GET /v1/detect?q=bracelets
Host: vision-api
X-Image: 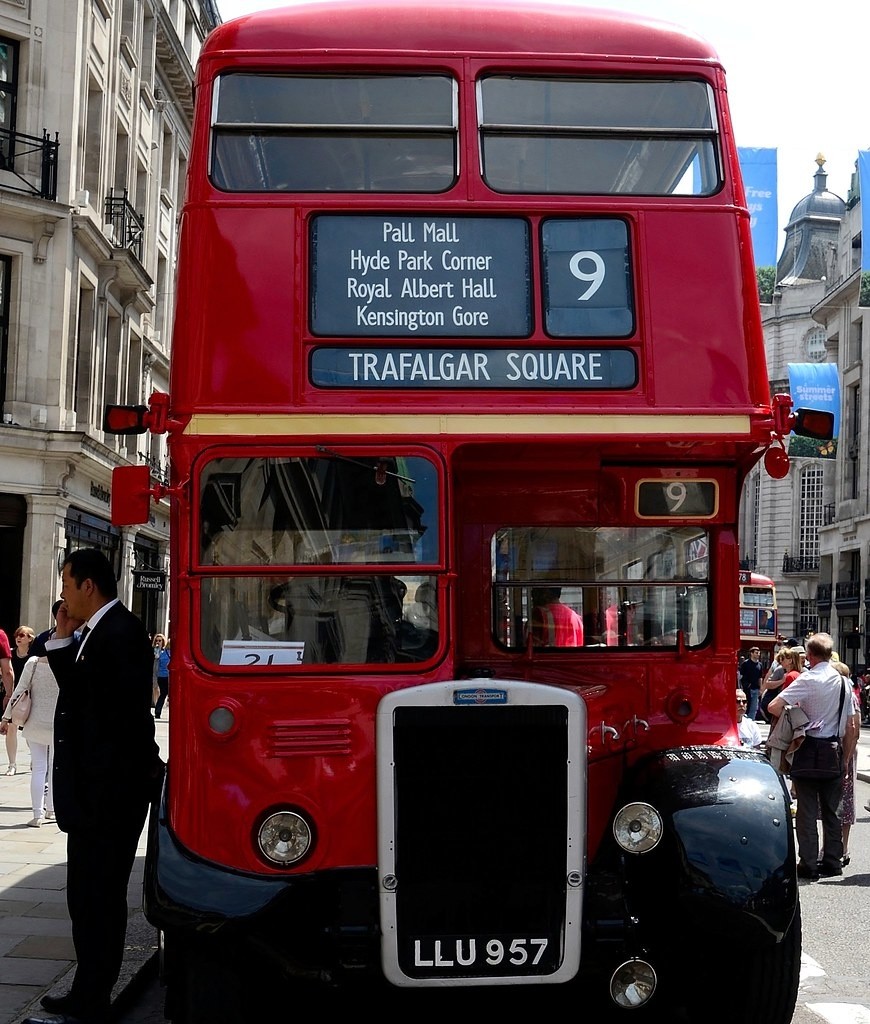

[6,694,11,698]
[2,719,9,722]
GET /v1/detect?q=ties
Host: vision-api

[74,625,91,661]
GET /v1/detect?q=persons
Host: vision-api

[523,588,583,646]
[607,589,635,645]
[0,600,83,827]
[404,583,438,632]
[40,547,170,1014]
[735,633,870,878]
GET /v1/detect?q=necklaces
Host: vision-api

[18,648,28,658]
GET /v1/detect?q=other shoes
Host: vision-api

[796,863,820,881]
[26,819,42,828]
[155,715,160,719]
[45,812,56,820]
[6,763,17,775]
[815,862,843,877]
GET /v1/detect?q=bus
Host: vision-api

[691,568,782,679]
[104,0,837,1024]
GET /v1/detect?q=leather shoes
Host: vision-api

[22,1017,68,1024]
[40,996,69,1015]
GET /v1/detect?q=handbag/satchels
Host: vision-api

[10,657,40,725]
[789,737,843,781]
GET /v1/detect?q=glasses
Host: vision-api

[14,632,29,638]
[736,700,748,705]
[777,657,785,662]
[155,640,163,642]
